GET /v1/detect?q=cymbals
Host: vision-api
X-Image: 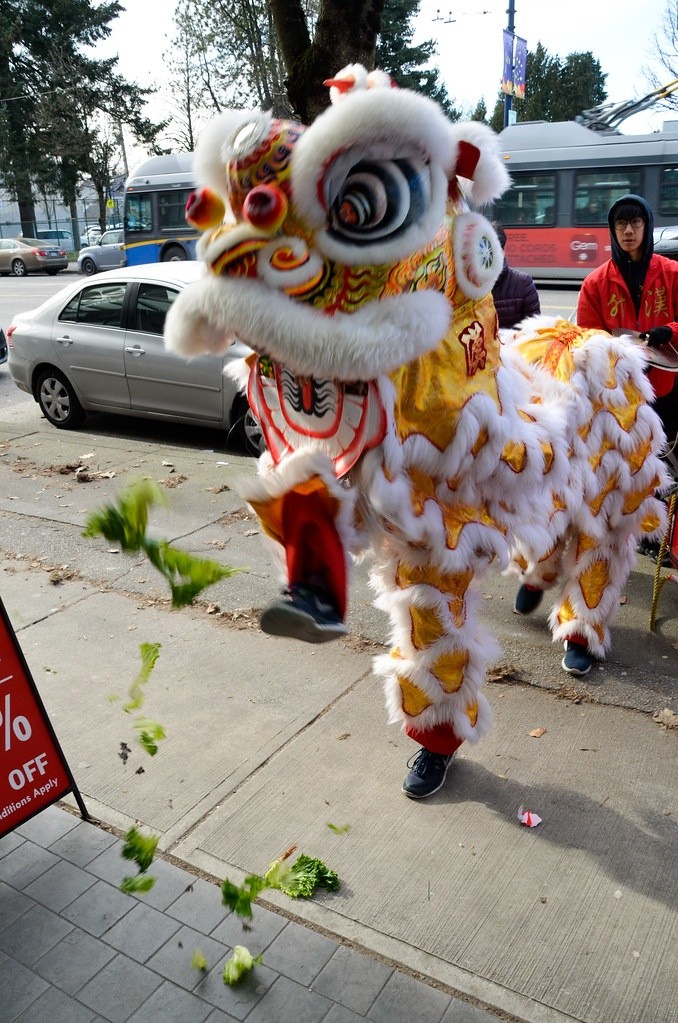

[612,327,677,373]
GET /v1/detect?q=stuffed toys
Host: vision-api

[160,64,674,807]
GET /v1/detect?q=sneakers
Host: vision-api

[402,745,459,799]
[515,584,544,616]
[262,592,347,644]
[561,639,591,675]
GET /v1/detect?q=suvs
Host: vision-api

[77,229,126,275]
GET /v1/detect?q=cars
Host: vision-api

[0,237,69,277]
[6,261,271,460]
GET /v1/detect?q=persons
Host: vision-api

[575,193,678,569]
[490,218,541,331]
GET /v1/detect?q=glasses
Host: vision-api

[614,219,645,231]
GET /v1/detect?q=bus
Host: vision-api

[476,117,677,290]
[123,150,203,265]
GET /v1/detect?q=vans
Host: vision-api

[19,229,89,252]
